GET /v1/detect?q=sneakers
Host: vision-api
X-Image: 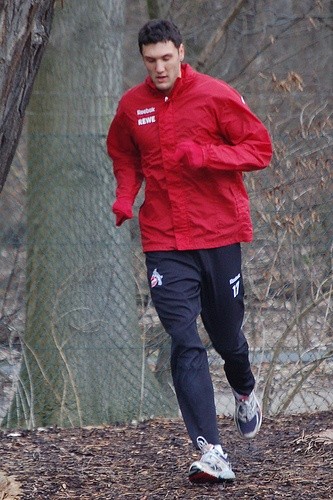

[189,436,235,482]
[230,385,263,440]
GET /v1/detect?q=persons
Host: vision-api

[106,20,274,480]
